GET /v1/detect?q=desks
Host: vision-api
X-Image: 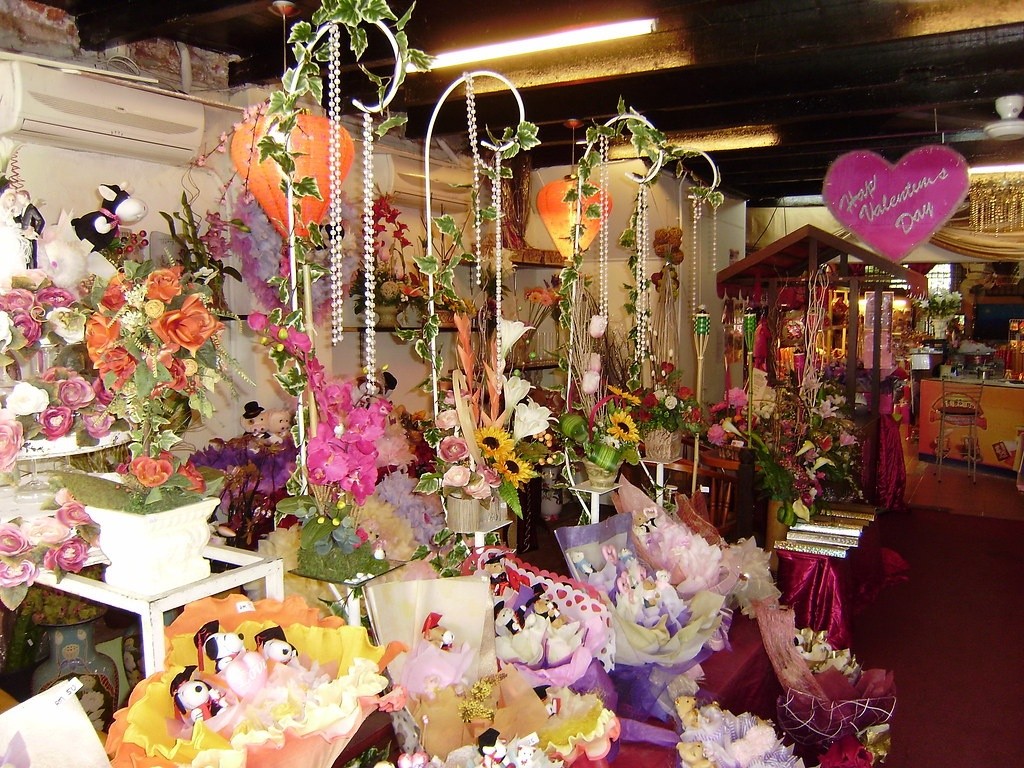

[917,373,1024,472]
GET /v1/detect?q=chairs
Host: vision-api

[933,374,985,484]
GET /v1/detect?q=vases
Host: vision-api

[446,496,481,533]
[121,607,181,690]
[30,608,119,736]
[644,425,680,461]
[930,317,949,337]
[83,496,222,598]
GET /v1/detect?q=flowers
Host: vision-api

[0,184,963,768]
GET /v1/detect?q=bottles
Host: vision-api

[974,348,981,366]
[984,353,991,365]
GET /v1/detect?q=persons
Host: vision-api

[1,190,45,271]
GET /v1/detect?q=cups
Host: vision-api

[983,372,989,379]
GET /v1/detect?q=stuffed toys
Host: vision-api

[676,696,720,768]
[573,546,671,605]
[792,628,847,672]
[422,613,454,652]
[71,182,148,252]
[356,371,397,409]
[169,618,299,725]
[485,554,565,635]
[476,728,538,768]
[239,401,294,443]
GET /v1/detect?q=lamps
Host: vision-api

[227,1,357,253]
[402,16,660,77]
[535,119,612,261]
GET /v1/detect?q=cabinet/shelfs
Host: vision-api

[31,540,284,679]
[327,253,570,379]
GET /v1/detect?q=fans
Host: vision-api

[895,96,1023,142]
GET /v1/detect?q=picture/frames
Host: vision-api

[582,456,623,487]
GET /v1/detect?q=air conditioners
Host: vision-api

[0,60,206,168]
